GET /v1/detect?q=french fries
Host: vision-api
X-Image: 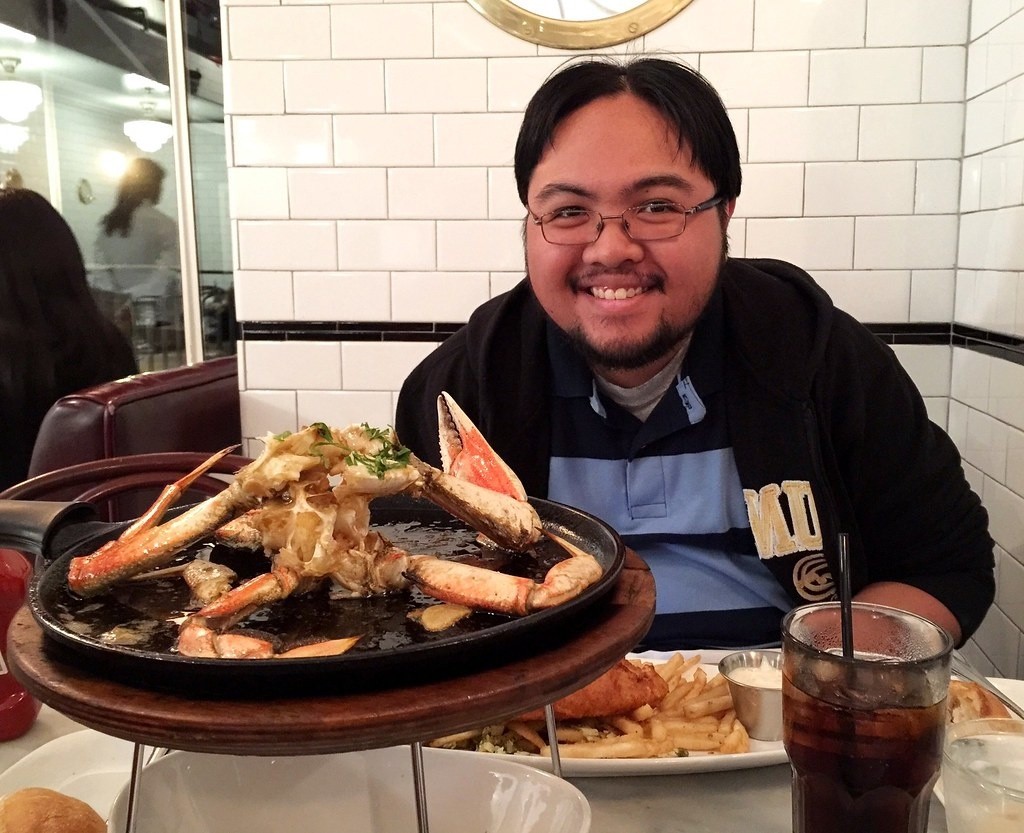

[433,653,754,759]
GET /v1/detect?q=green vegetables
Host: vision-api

[274,422,411,481]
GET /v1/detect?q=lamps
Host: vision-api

[0,56,43,123]
[123,103,173,153]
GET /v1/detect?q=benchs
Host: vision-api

[27,353,243,524]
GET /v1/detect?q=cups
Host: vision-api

[780,599,955,833]
[939,716,1024,833]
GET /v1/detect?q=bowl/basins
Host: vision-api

[718,650,785,742]
[107,745,592,833]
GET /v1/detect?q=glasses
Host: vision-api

[530,196,723,245]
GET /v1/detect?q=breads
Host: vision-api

[942,678,1019,735]
[0,787,108,833]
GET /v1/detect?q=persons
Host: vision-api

[1,186,140,487]
[92,159,179,323]
[396,59,996,663]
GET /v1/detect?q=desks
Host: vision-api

[0,675,1023,833]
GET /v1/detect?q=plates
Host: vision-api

[464,656,790,778]
[0,728,177,833]
[28,495,627,704]
[949,675,1024,721]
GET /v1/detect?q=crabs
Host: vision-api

[64,388,604,660]
